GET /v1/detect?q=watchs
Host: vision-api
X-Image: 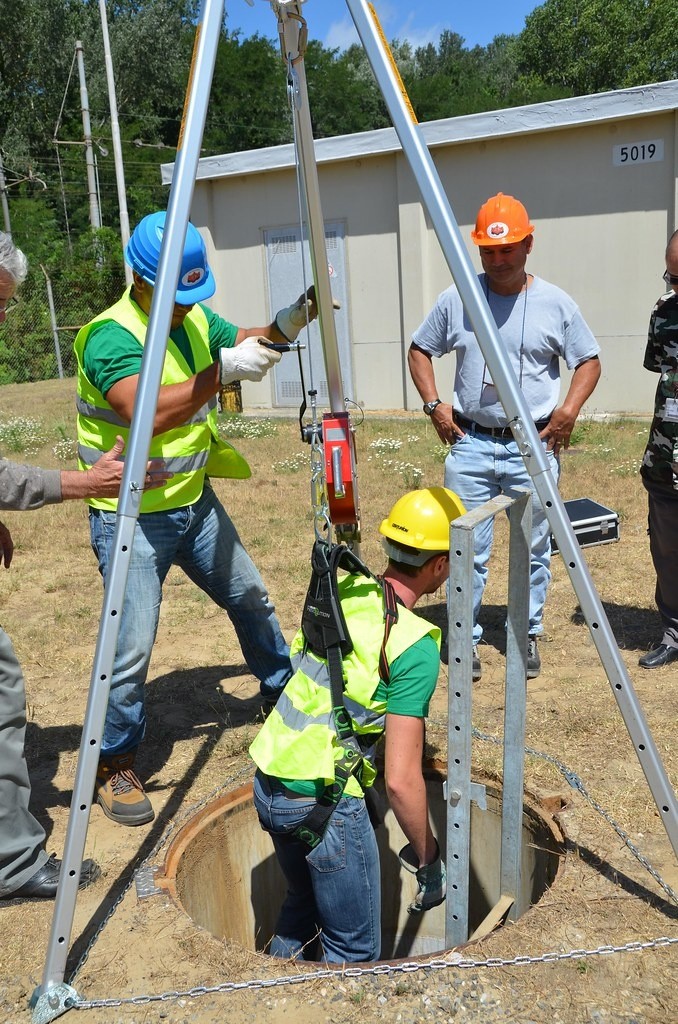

[423,399,442,415]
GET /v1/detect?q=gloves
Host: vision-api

[399,836,446,914]
[275,284,340,343]
[219,335,283,385]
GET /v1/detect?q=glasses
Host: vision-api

[482,343,524,387]
[0,296,19,314]
[663,269,678,285]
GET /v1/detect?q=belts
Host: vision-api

[453,413,550,438]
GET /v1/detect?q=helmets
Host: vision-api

[124,211,216,306]
[471,192,535,247]
[380,487,476,554]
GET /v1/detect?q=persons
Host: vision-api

[246,489,470,968]
[406,191,601,679]
[0,227,174,909]
[71,212,343,826]
[638,230,678,669]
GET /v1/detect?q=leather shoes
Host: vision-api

[639,643,678,668]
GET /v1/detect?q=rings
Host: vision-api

[556,441,561,445]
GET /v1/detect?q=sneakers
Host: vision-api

[93,745,155,827]
[526,634,541,678]
[0,853,101,907]
[262,700,277,716]
[472,652,482,678]
